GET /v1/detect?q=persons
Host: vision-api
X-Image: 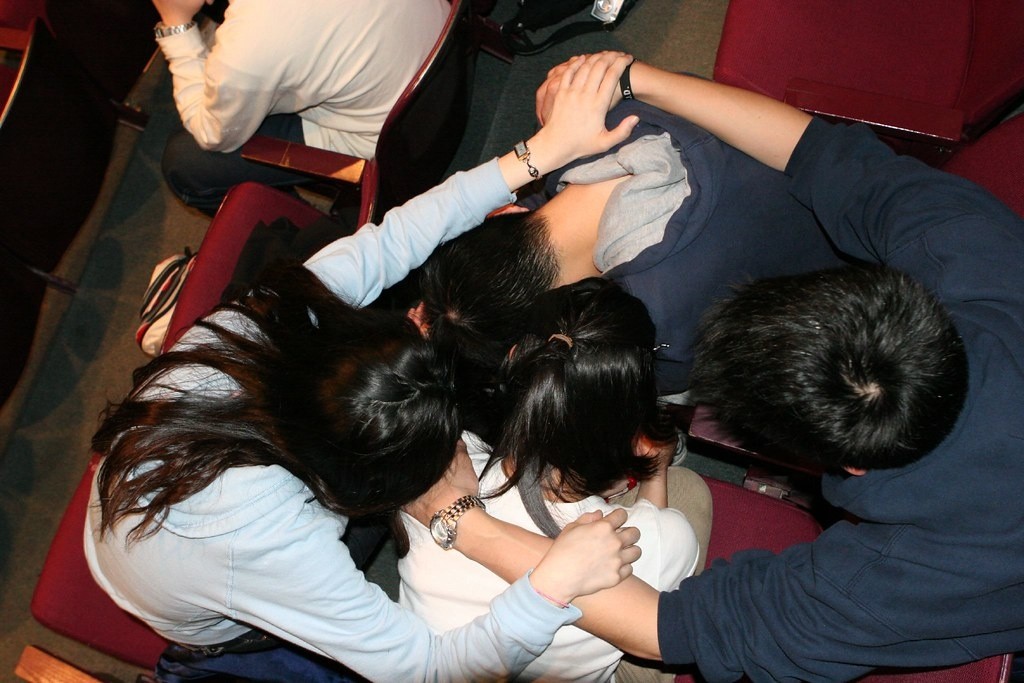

[152,1,451,220]
[83,49,1024,683]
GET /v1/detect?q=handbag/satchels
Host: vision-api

[135,244,203,359]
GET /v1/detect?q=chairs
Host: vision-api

[1,1,1024,683]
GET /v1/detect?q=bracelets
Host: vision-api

[619,58,638,101]
[536,589,571,608]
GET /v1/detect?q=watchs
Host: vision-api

[154,22,195,38]
[430,494,487,550]
[514,140,542,181]
